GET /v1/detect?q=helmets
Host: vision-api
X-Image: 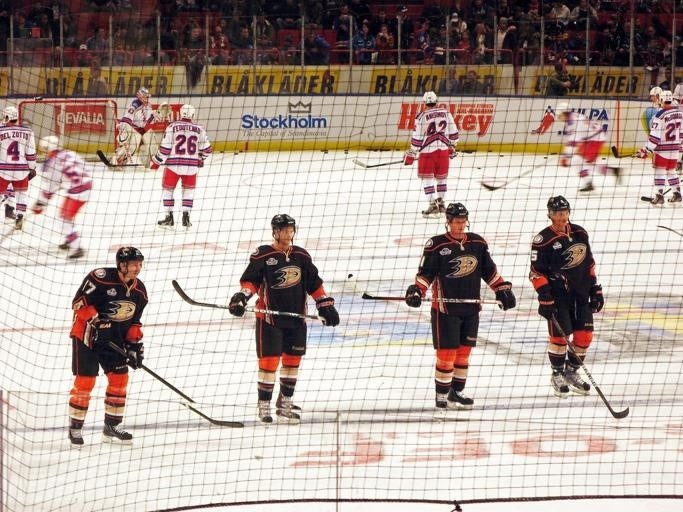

[135,86,151,107]
[1,105,19,124]
[116,246,144,274]
[554,102,574,120]
[650,86,673,106]
[445,202,469,223]
[422,91,438,104]
[546,195,570,211]
[39,136,63,152]
[271,213,296,244]
[180,104,196,119]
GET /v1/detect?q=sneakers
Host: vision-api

[69,428,84,444]
[550,366,569,392]
[448,388,473,405]
[258,400,272,423]
[103,423,132,440]
[563,362,590,391]
[614,167,623,184]
[182,212,192,226]
[435,392,448,407]
[579,182,594,192]
[650,186,682,204]
[276,392,300,409]
[5,204,23,230]
[158,212,174,226]
[59,241,84,258]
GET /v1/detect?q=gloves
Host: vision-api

[405,284,422,307]
[229,292,246,316]
[124,340,144,370]
[402,149,416,165]
[31,200,47,214]
[493,281,516,311]
[91,321,113,347]
[538,292,559,319]
[589,284,604,312]
[150,163,160,169]
[316,297,339,327]
[449,145,456,159]
[633,146,653,159]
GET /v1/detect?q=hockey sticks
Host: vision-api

[352,159,420,168]
[642,188,671,202]
[611,146,633,158]
[107,340,243,427]
[348,273,503,304]
[172,280,326,321]
[96,150,146,167]
[479,155,559,191]
[551,312,630,418]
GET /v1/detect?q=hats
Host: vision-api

[79,44,88,50]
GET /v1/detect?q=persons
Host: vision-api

[1,183,17,220]
[229,213,340,414]
[554,101,624,193]
[631,70,683,203]
[1,0,682,71]
[437,69,458,93]
[29,134,93,260]
[63,245,149,447]
[0,105,37,230]
[526,195,606,393]
[111,85,153,172]
[542,60,572,97]
[462,71,484,94]
[404,202,517,410]
[401,90,461,214]
[148,103,213,228]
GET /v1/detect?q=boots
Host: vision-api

[422,198,446,215]
